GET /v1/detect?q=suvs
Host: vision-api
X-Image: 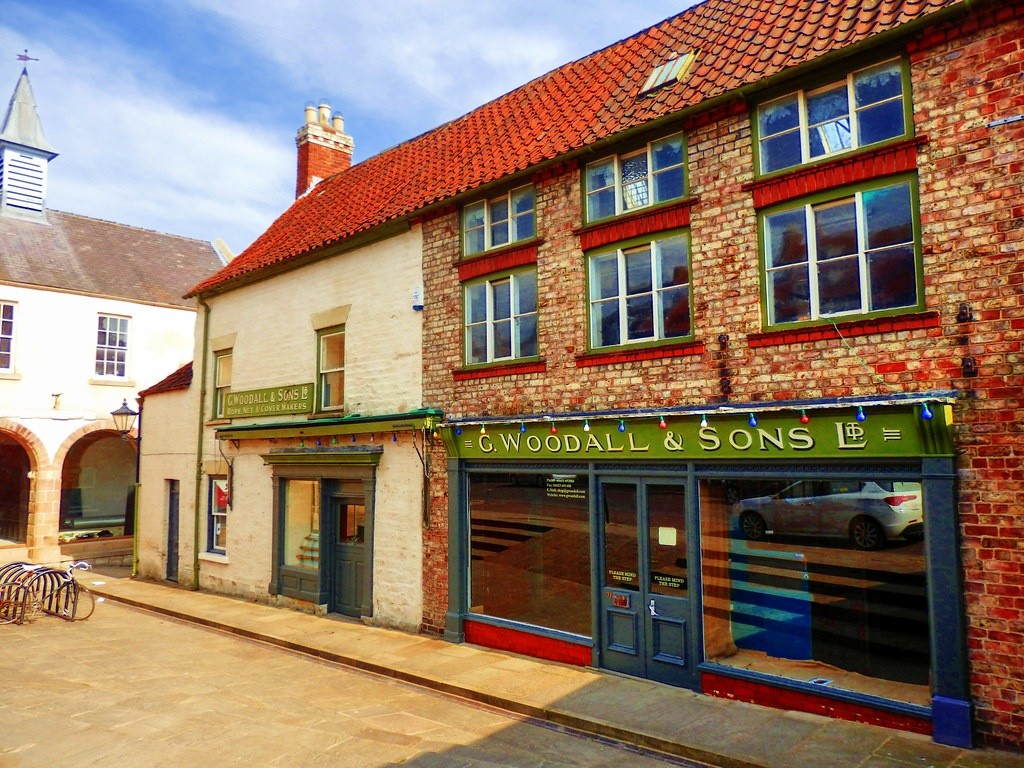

[728,478,924,552]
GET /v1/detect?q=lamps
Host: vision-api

[112,399,142,443]
